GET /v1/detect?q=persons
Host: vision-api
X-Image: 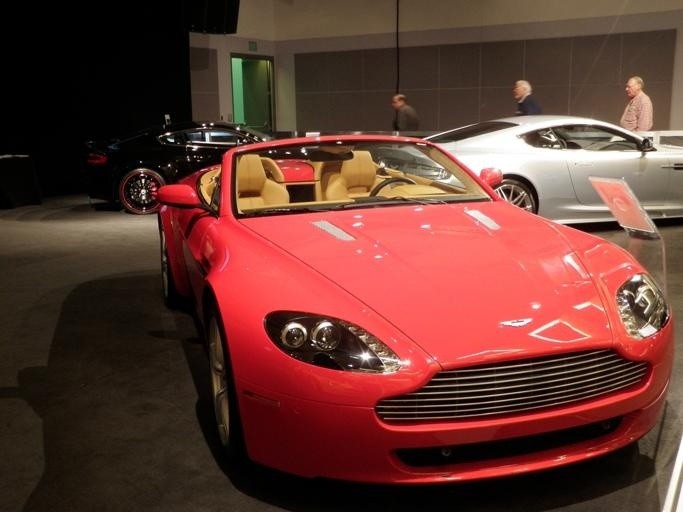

[620,76,655,132]
[513,80,543,116]
[391,94,420,131]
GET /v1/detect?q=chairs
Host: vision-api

[202,152,289,209]
[322,150,394,201]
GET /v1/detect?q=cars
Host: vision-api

[72,119,283,219]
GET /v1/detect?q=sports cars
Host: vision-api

[416,112,683,231]
[149,132,674,491]
